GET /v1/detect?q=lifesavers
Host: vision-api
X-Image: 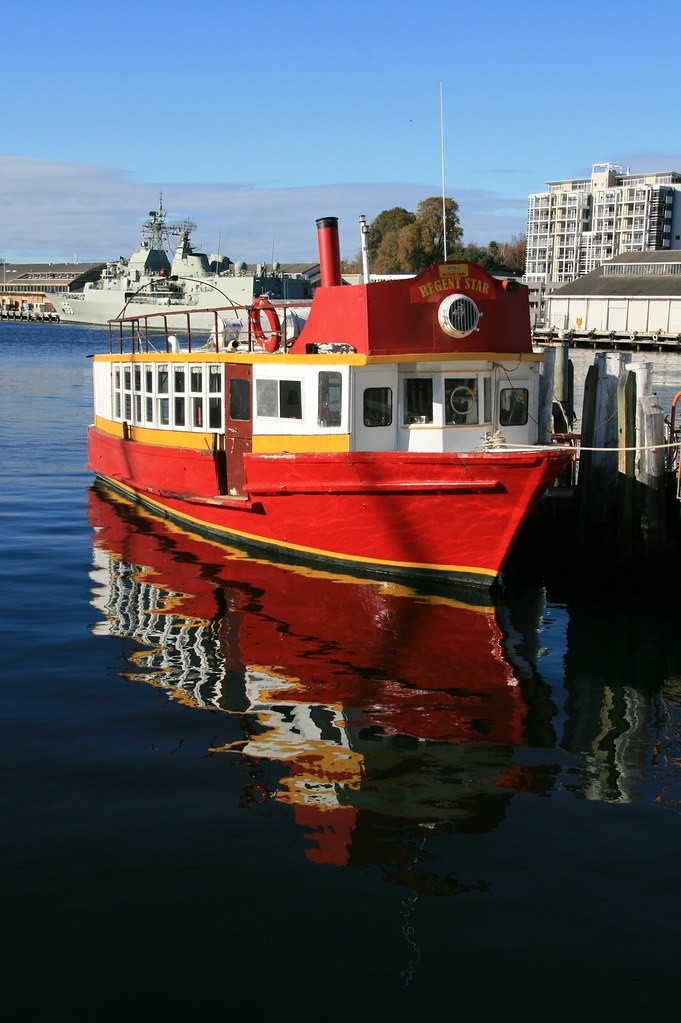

[249,295,283,353]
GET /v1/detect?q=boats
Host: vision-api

[82,86,577,589]
[43,189,291,335]
[87,475,564,866]
